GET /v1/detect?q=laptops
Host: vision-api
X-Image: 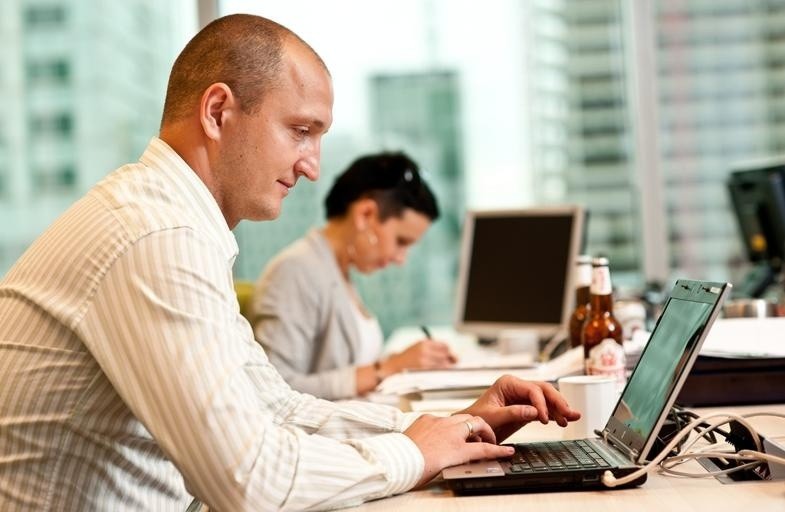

[440,278,733,497]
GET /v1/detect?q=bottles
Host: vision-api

[569,254,626,381]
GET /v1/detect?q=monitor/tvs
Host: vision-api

[727,166,785,302]
[449,205,583,358]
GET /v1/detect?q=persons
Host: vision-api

[243,148,462,405]
[1,12,584,511]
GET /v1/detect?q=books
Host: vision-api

[368,321,583,398]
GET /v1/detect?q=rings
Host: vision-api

[463,419,475,440]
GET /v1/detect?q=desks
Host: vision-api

[194,358,785,511]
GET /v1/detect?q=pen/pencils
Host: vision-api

[422,326,432,340]
